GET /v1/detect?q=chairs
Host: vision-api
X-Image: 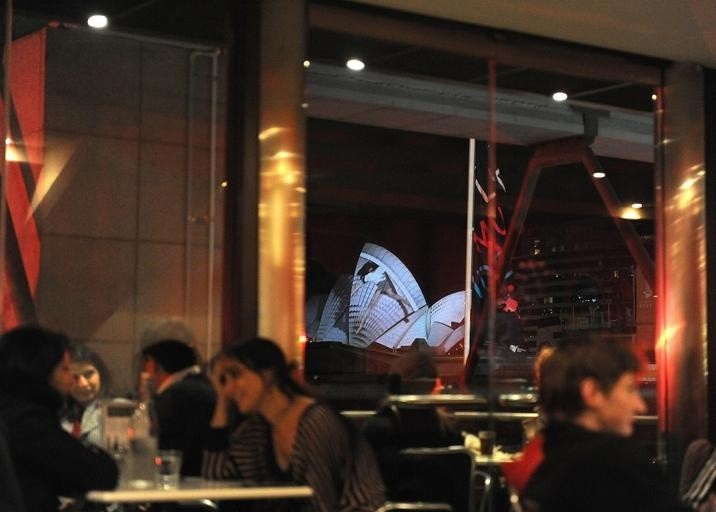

[376,447,490,511]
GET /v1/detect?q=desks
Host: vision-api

[82,478,317,511]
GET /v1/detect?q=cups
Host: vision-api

[153,454,181,489]
[477,430,498,456]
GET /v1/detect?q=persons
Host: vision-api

[194,332,387,510]
[353,350,469,501]
[516,331,673,512]
[57,338,110,446]
[495,344,564,492]
[0,323,118,510]
[136,317,249,481]
